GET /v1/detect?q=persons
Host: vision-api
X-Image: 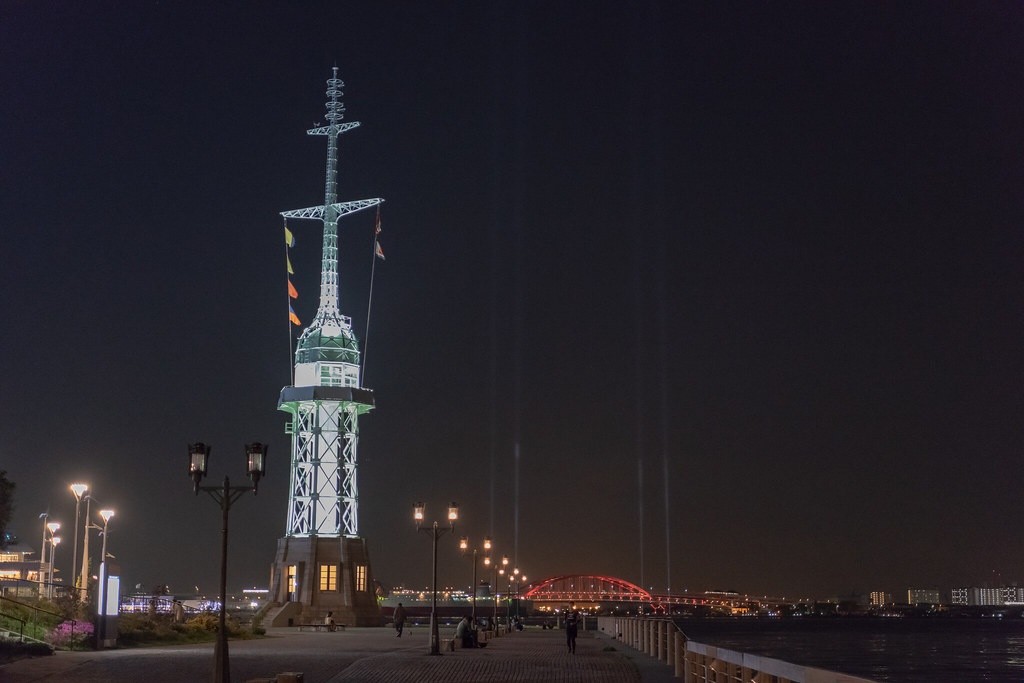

[393,602,407,638]
[170,599,183,622]
[325,611,336,632]
[456,616,481,648]
[563,601,581,655]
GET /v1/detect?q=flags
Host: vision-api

[288,281,298,298]
[288,260,294,274]
[285,228,292,248]
[289,305,301,325]
[375,241,385,258]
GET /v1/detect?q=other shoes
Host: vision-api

[568,648,571,653]
[396,633,402,639]
[331,630,337,632]
[572,651,575,655]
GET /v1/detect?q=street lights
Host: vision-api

[413,501,459,656]
[70,484,88,599]
[510,575,527,627]
[97,510,115,651]
[187,440,269,683]
[460,535,491,649]
[498,568,519,633]
[47,523,61,601]
[483,553,509,639]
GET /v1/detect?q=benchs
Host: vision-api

[439,624,508,652]
[298,623,347,632]
